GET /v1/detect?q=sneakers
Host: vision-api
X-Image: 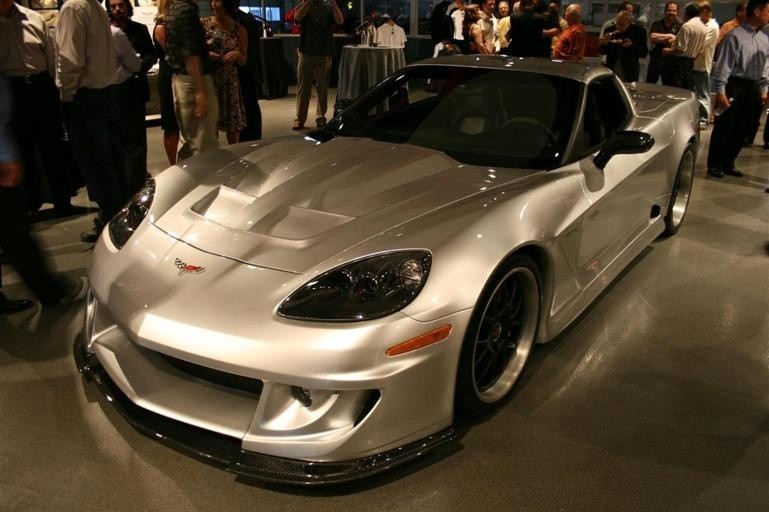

[61,274,89,306]
[293,120,304,129]
[80,216,102,242]
[316,118,326,128]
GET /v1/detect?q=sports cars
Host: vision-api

[72,51,704,489]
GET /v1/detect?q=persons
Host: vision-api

[599,2,648,83]
[293,1,344,129]
[646,2,719,129]
[1,1,157,316]
[425,1,587,92]
[708,1,769,177]
[153,0,262,165]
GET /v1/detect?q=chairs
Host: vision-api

[439,73,604,146]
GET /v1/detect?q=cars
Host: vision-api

[142,60,162,115]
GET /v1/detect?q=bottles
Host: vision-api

[367,20,378,45]
[361,30,367,45]
[353,31,360,46]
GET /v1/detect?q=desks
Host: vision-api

[336,44,406,116]
[259,36,289,99]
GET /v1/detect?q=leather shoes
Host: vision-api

[707,165,724,179]
[725,167,744,178]
[1,293,33,313]
